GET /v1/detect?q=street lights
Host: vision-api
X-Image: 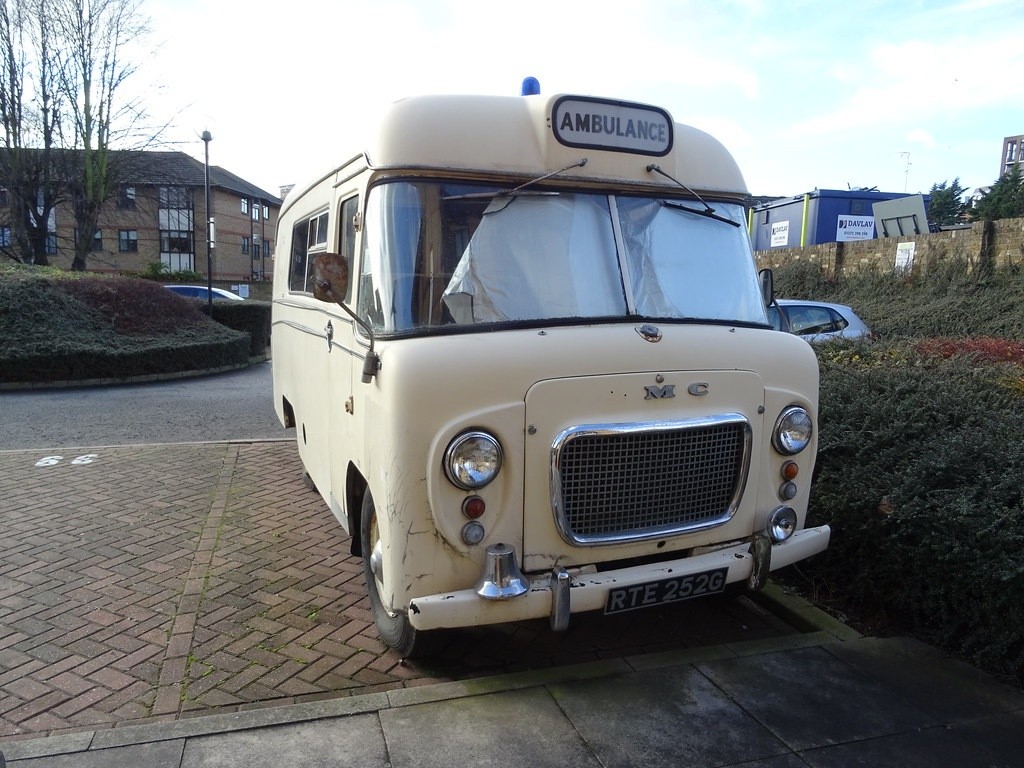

[201,131,213,319]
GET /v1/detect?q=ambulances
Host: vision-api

[269,76,832,662]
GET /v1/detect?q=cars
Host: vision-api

[765,299,873,344]
[164,285,244,301]
